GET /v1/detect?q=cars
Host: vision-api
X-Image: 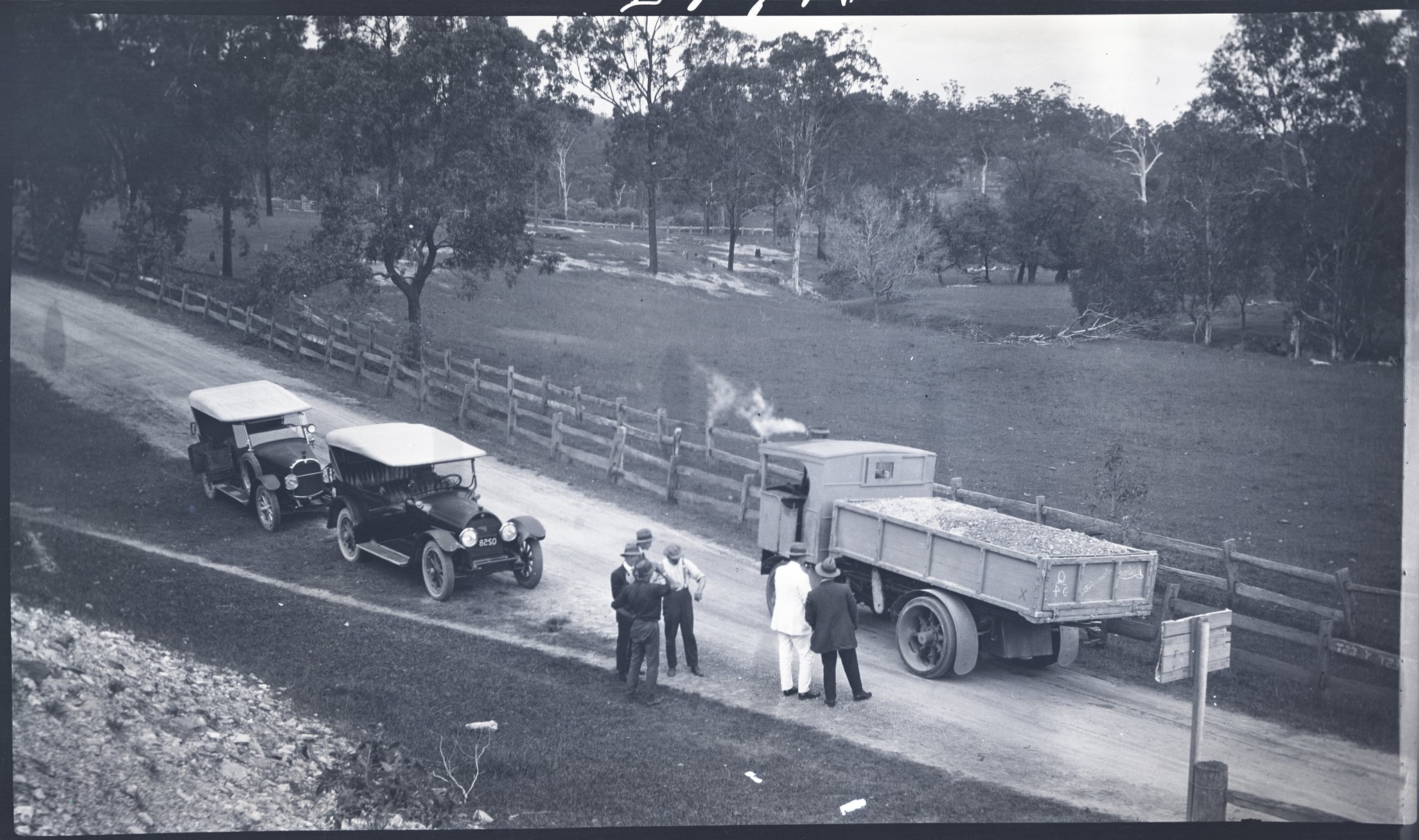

[323,423,546,602]
[190,379,336,531]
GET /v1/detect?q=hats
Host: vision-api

[633,561,658,573]
[635,528,656,541]
[622,543,643,556]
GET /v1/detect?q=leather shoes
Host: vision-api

[814,556,842,577]
[854,691,872,700]
[824,698,835,706]
[663,543,686,560]
[798,690,820,700]
[784,687,799,696]
[638,670,645,674]
[691,666,704,676]
[648,697,665,705]
[619,675,628,682]
[667,666,676,676]
[788,542,812,556]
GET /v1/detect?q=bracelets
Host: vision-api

[662,572,668,577]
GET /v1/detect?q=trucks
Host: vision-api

[758,426,1162,680]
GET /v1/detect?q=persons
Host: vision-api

[610,560,679,705]
[609,543,646,681]
[633,528,658,662]
[656,544,707,676]
[768,542,820,700]
[804,556,872,707]
